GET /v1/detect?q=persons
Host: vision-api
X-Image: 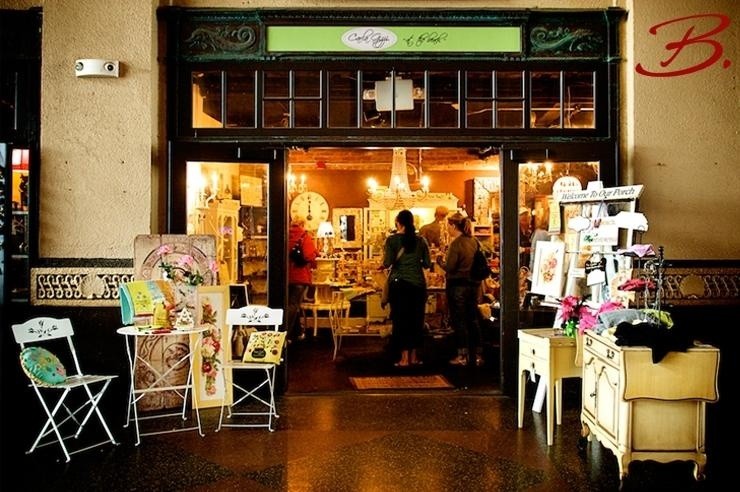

[288,214,321,344]
[436,213,492,368]
[519,207,532,271]
[529,211,551,273]
[419,206,449,247]
[383,210,431,369]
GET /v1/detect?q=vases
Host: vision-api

[562,318,577,337]
[173,303,195,331]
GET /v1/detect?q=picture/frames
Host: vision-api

[331,207,363,250]
[239,174,264,208]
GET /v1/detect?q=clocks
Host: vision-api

[290,191,331,232]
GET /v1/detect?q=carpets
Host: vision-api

[348,374,455,391]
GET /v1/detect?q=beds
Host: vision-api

[329,301,454,360]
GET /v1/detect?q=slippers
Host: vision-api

[394,362,410,367]
[411,361,424,365]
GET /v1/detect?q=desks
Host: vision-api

[116,325,213,447]
[299,302,351,341]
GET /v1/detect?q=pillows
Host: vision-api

[18,346,67,386]
[241,330,287,365]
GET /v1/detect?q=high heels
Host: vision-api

[450,355,468,366]
[475,356,485,366]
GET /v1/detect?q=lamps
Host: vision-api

[315,221,335,258]
[364,148,431,212]
[519,160,554,199]
[287,165,307,194]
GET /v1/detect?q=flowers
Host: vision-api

[155,226,235,305]
[556,293,592,335]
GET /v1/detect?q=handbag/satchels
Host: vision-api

[471,238,492,281]
[289,231,310,268]
[382,247,404,309]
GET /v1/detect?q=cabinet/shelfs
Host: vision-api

[574,323,720,492]
[366,291,392,320]
[464,175,525,225]
[471,224,494,260]
[196,204,239,285]
[516,326,585,447]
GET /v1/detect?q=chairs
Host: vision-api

[213,304,284,435]
[10,316,121,465]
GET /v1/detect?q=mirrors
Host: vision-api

[364,192,461,261]
